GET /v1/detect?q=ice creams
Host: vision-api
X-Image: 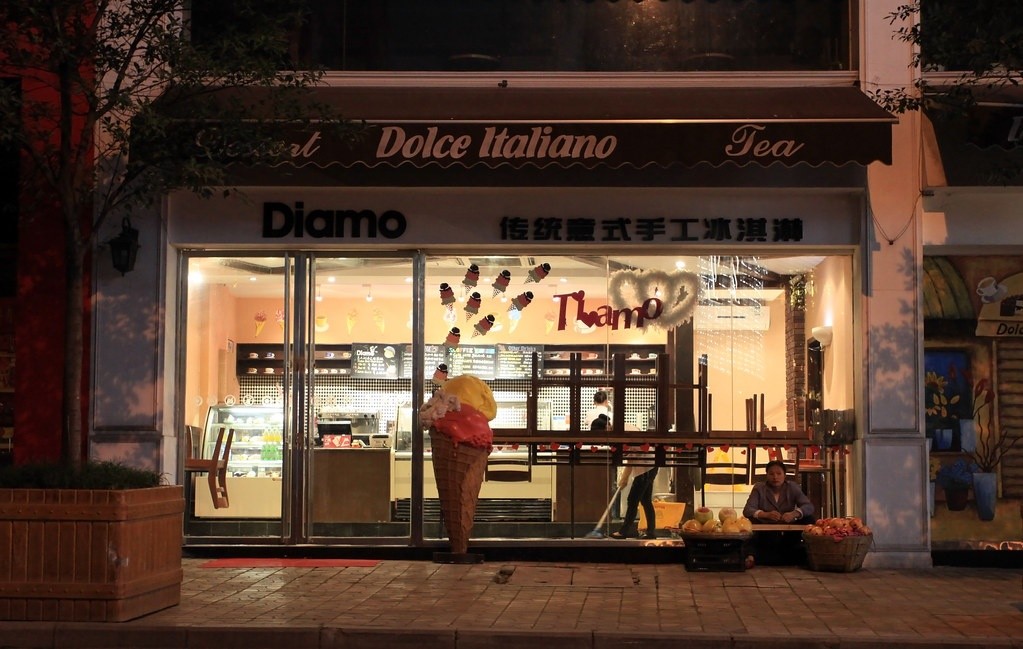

[419,374,497,554]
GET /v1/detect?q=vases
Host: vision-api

[935,428,952,450]
[927,437,933,452]
[974,472,999,522]
[929,481,935,516]
[944,485,965,510]
[958,418,977,452]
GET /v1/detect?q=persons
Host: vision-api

[586,391,613,426]
[743,461,815,569]
[591,414,658,538]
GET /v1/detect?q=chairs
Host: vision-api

[184,426,235,510]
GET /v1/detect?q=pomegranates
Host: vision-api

[804,517,871,535]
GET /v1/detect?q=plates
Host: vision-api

[265,357,274,358]
[981,285,1008,304]
[316,325,329,332]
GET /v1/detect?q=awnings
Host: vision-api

[126,86,899,166]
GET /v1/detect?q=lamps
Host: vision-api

[109,214,140,277]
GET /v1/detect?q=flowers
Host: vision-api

[925,370,1023,490]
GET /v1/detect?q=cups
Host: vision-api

[588,353,598,357]
[314,369,347,373]
[265,367,274,372]
[266,352,275,357]
[586,369,603,374]
[976,277,999,297]
[326,353,334,357]
[250,353,258,357]
[632,369,641,373]
[629,353,657,359]
[249,368,257,372]
[316,316,327,326]
[343,353,351,357]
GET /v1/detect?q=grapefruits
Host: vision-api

[682,506,752,533]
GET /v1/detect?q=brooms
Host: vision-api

[583,486,622,539]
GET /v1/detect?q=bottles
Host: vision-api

[274,426,281,442]
[262,443,283,460]
[263,426,269,442]
[269,427,274,442]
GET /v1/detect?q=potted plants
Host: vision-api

[1,454,184,623]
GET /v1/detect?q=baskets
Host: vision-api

[799,531,874,572]
[676,529,756,572]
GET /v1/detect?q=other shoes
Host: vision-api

[638,533,657,539]
[604,532,626,539]
